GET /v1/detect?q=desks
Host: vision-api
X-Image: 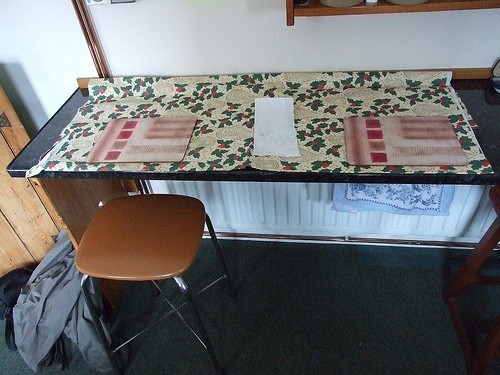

[8,79,500,316]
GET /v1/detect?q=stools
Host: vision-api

[74,195,236,375]
[443,183,500,375]
